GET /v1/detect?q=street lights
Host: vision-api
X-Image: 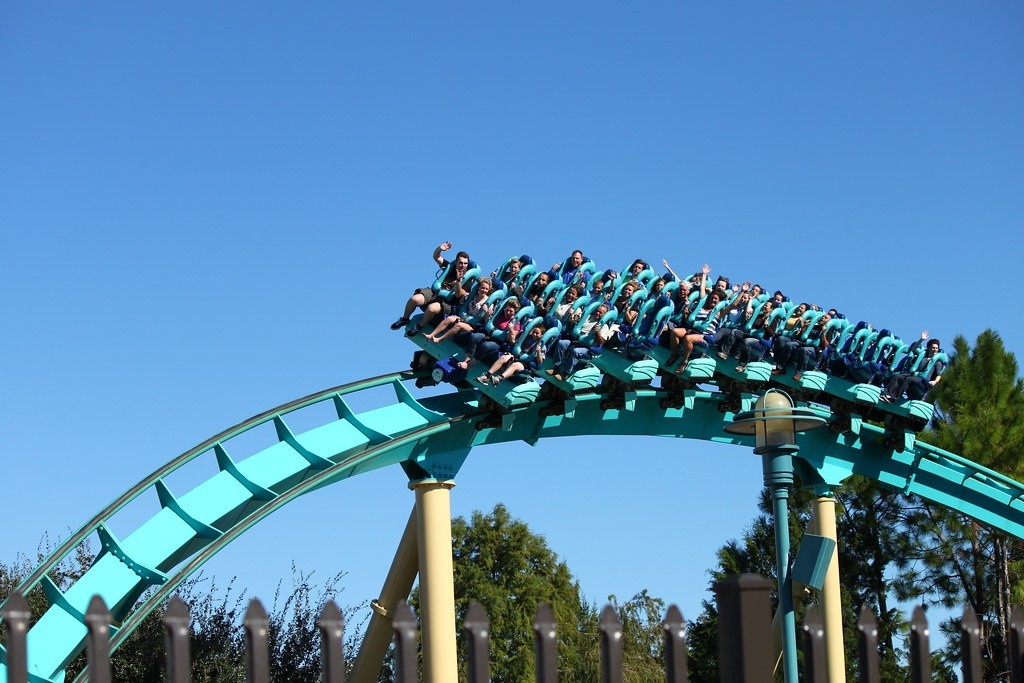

[721,386,836,683]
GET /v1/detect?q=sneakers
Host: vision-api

[407,325,421,337]
[391,317,410,330]
[491,375,499,387]
[476,375,490,386]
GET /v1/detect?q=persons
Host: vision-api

[390,241,950,406]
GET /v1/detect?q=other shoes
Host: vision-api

[554,373,568,381]
[735,354,741,360]
[675,361,688,374]
[793,374,800,381]
[545,367,560,375]
[458,358,471,371]
[879,393,896,404]
[735,365,746,373]
[716,352,727,360]
[665,353,680,367]
[772,367,785,375]
